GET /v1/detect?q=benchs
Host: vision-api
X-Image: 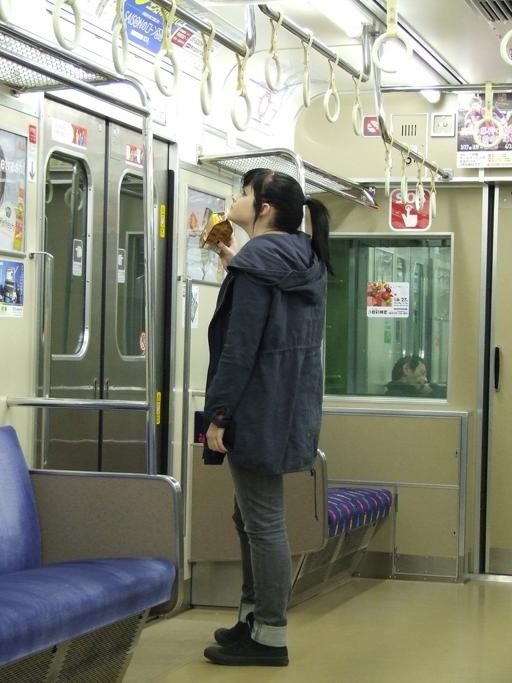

[193,411,392,610]
[0,427,184,683]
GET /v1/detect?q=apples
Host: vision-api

[381,291,393,306]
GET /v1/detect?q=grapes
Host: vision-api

[384,284,391,292]
[367,282,384,306]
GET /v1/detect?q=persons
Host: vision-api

[385,355,440,397]
[202,167,336,668]
[465,97,495,141]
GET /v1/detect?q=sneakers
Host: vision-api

[213,622,250,646]
[204,640,289,666]
